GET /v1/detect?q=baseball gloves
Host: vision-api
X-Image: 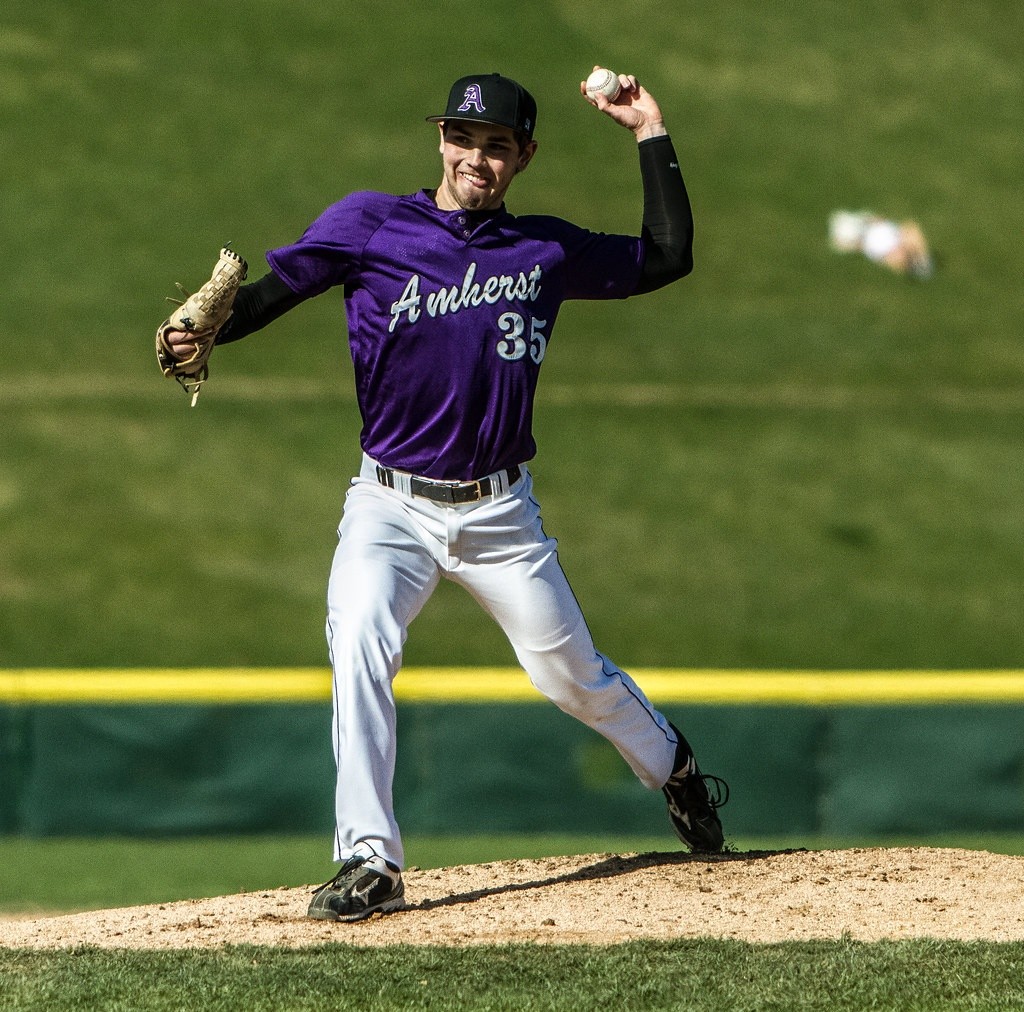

[154,243,250,408]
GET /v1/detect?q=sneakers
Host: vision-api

[662,721,730,855]
[307,841,407,922]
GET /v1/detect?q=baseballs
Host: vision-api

[585,68,622,104]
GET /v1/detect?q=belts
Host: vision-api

[377,465,522,503]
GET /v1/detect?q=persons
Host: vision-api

[830,207,933,276]
[156,65,730,921]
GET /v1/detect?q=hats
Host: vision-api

[426,72,537,142]
